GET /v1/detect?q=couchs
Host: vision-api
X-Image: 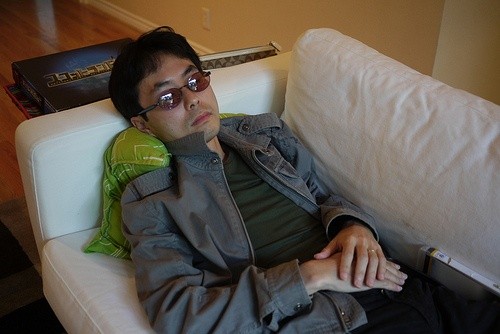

[15,28,500,334]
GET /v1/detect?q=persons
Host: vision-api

[108,25,500,334]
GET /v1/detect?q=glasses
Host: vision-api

[137,71,211,116]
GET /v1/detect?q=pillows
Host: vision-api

[83,113,257,262]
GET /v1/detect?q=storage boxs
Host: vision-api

[11,37,136,113]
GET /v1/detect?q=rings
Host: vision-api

[368,248,376,252]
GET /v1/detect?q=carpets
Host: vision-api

[0,222,70,334]
